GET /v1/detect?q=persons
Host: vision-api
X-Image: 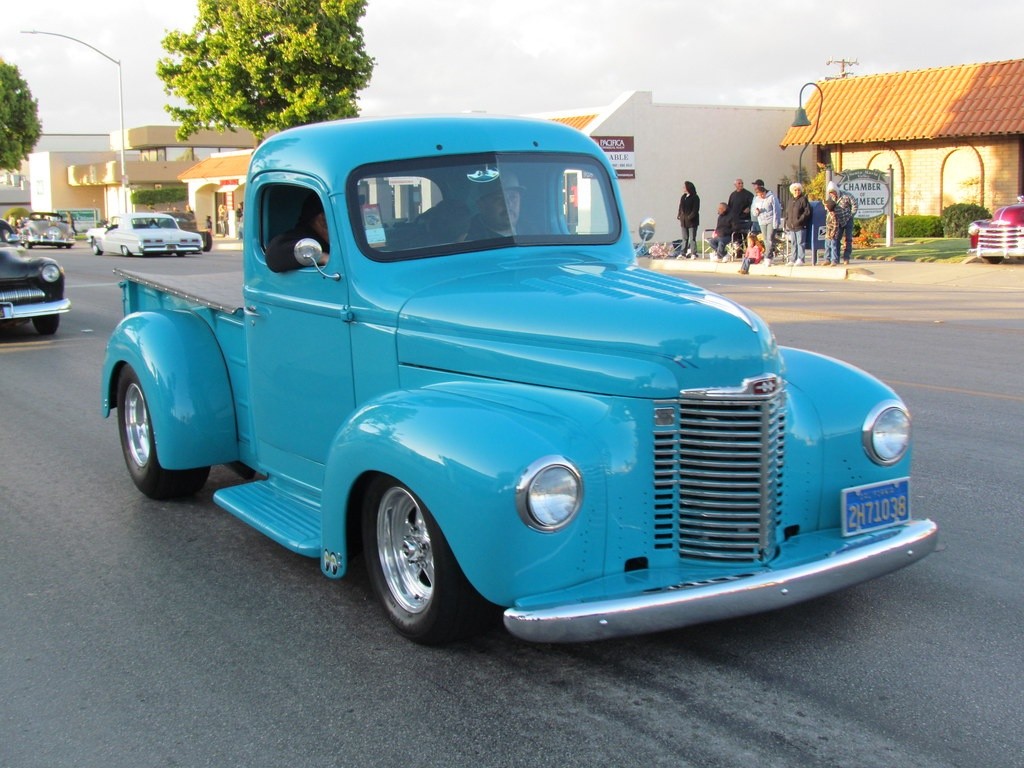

[826,181,858,265]
[783,183,812,266]
[66,210,77,236]
[146,201,244,241]
[737,236,761,274]
[727,178,754,258]
[264,186,366,273]
[754,187,781,267]
[749,178,765,235]
[708,201,735,263]
[676,181,700,260]
[459,167,528,242]
[820,200,842,266]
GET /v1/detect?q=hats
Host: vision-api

[752,179,764,186]
[824,200,836,211]
[479,170,527,201]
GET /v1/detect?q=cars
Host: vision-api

[967,193,1024,263]
[18,212,78,248]
[0,219,72,334]
[85,213,205,256]
[160,211,212,251]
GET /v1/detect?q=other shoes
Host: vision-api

[764,258,773,267]
[739,269,748,274]
[844,258,849,265]
[722,253,732,262]
[785,259,804,266]
[691,255,696,260]
[677,254,686,259]
[821,260,837,266]
[710,255,720,261]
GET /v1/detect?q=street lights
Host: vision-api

[791,82,824,183]
[19,29,126,210]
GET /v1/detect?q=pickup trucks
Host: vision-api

[101,110,935,644]
